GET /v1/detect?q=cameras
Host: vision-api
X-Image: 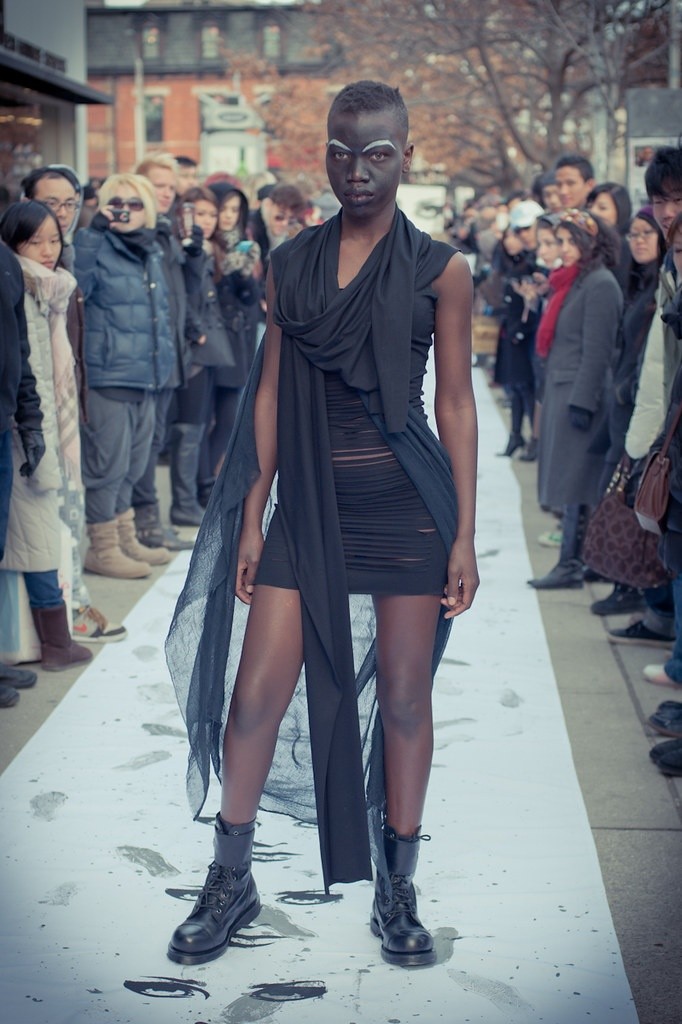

[108,210,130,223]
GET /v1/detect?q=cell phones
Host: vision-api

[231,241,253,254]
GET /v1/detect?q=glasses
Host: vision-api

[40,198,77,213]
[624,228,657,240]
[274,212,298,227]
[108,196,145,211]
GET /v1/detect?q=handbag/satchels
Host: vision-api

[581,449,667,589]
[634,452,670,536]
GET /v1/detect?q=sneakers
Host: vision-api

[72,605,127,642]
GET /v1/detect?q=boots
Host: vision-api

[33,602,92,672]
[82,508,171,578]
[168,809,260,963]
[170,422,206,525]
[370,821,436,966]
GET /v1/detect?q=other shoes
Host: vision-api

[503,431,524,455]
[643,664,677,687]
[1,665,37,706]
[538,530,563,548]
[518,437,538,462]
[527,568,583,589]
[138,523,194,551]
[591,594,647,615]
[649,738,681,777]
[648,699,682,738]
[608,621,676,648]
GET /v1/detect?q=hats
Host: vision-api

[510,201,545,230]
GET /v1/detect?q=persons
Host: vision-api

[166,81,481,967]
[0,156,343,711]
[443,148,682,774]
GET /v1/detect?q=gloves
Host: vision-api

[18,428,47,477]
[568,406,592,431]
